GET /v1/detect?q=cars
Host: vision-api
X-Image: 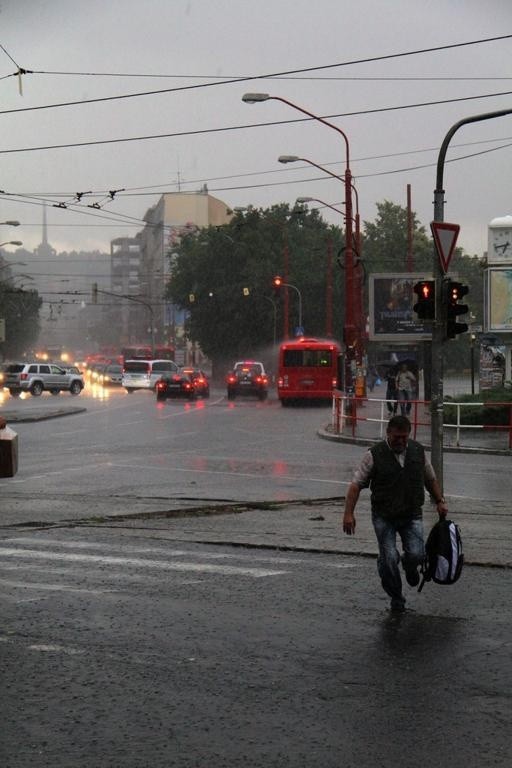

[0,359,85,397]
[227,367,265,400]
[156,366,210,403]
[73,355,124,387]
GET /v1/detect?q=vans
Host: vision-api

[121,358,181,393]
[234,362,268,396]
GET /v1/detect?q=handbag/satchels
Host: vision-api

[427,520,463,585]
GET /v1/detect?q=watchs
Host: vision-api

[436,495,446,504]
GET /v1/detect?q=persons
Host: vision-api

[0,415,8,430]
[342,414,449,611]
[365,358,417,416]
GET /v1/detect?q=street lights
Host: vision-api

[277,155,366,410]
[272,278,304,337]
[240,93,360,429]
[295,196,369,403]
[241,291,277,385]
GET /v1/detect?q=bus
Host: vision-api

[121,343,176,361]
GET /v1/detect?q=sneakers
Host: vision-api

[390,597,406,613]
[401,553,419,586]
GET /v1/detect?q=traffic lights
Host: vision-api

[444,278,471,341]
[411,277,436,322]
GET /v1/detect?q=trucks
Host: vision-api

[44,342,62,359]
